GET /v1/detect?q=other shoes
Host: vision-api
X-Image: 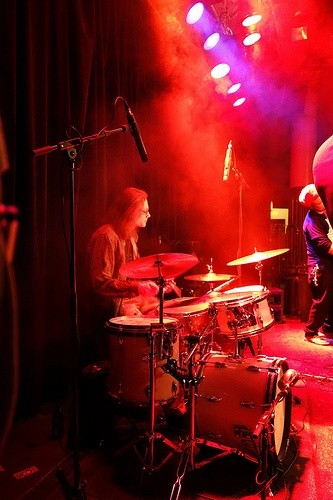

[304,330,333,346]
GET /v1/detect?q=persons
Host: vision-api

[298,185,333,345]
[88,188,178,379]
[312,134,333,226]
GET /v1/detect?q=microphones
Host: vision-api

[122,101,148,163]
[222,144,232,182]
[279,367,297,390]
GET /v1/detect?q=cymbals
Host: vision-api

[184,274,239,281]
[119,253,198,279]
[227,247,290,266]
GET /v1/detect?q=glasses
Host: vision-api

[139,209,150,215]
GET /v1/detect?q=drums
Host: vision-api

[155,296,216,337]
[211,284,275,340]
[194,350,292,466]
[104,315,180,404]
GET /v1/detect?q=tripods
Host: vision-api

[125,279,223,488]
